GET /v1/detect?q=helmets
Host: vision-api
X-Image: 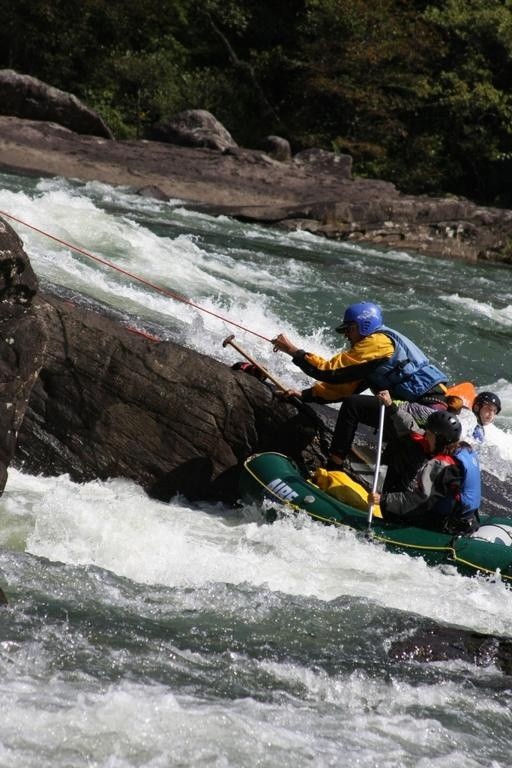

[472,392,501,414]
[425,411,461,446]
[335,303,383,336]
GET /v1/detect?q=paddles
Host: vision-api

[223,334,376,473]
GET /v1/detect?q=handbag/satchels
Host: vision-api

[447,396,463,414]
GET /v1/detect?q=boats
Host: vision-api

[241,450,512,586]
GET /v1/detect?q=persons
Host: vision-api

[367,387,484,540]
[472,389,502,443]
[270,298,454,495]
